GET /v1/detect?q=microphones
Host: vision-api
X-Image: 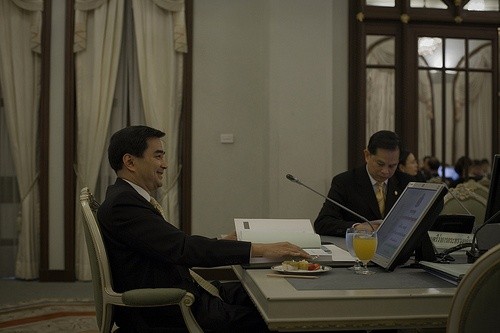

[439,182,471,215]
[286,174,375,231]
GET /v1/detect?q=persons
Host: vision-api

[314,130,427,239]
[395,148,492,188]
[96,125,311,333]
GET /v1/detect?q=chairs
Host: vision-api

[80,187,205,333]
[441,178,491,232]
[446,245,500,333]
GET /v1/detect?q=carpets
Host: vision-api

[0,296,119,333]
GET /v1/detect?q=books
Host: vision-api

[232,216,332,270]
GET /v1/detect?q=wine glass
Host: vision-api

[345,227,371,273]
[352,229,378,274]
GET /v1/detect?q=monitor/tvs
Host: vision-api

[438,165,459,180]
[371,181,448,272]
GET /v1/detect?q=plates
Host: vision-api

[270,265,333,273]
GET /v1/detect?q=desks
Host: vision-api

[219,234,457,333]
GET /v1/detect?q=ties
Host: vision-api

[150,196,223,301]
[375,182,385,219]
